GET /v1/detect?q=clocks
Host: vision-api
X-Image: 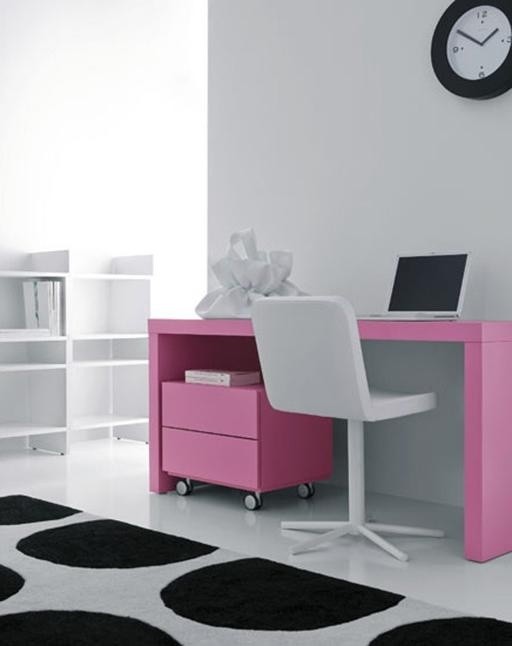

[430,0,512,100]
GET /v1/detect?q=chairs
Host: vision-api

[249,296,446,564]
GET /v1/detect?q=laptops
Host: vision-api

[356,250,471,321]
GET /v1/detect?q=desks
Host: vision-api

[148,318,511,563]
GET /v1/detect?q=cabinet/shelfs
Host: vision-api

[0,271,155,458]
[160,380,334,512]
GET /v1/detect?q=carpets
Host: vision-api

[0,496,511,646]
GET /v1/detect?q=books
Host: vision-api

[183,368,262,387]
[0,279,61,339]
